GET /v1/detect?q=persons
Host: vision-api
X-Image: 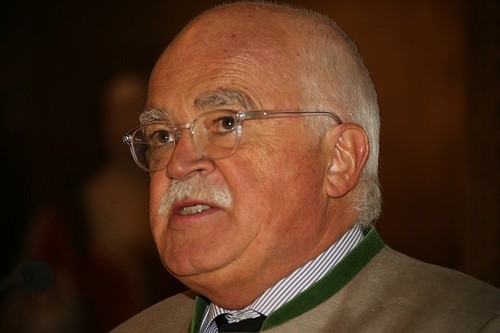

[108,4,500,332]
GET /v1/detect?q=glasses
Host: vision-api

[122,108,343,176]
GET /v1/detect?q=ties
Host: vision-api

[215,313,267,333]
